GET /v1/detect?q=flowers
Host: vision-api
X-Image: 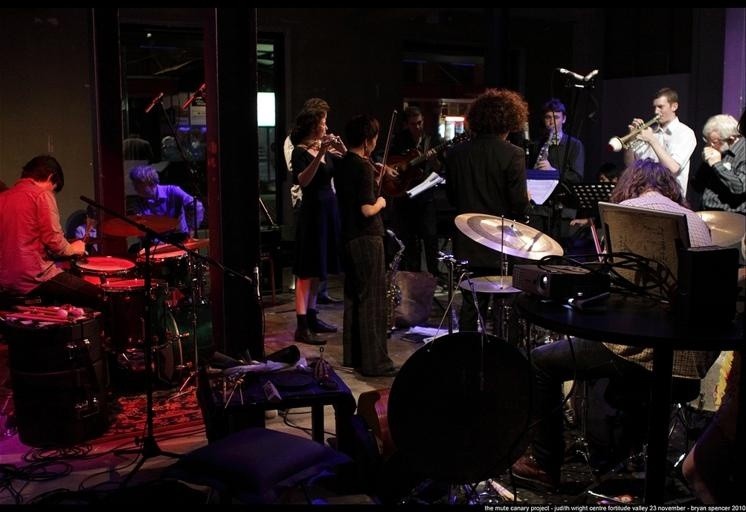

[179,83,206,111]
[253,266,262,301]
[144,92,164,113]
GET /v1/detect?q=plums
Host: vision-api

[511,264,611,300]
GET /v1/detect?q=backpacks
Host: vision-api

[143,296,209,393]
[493,299,522,327]
[136,238,195,310]
[102,279,168,359]
[3,306,110,400]
[74,255,140,285]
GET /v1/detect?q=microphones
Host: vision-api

[1,291,54,415]
[261,254,276,305]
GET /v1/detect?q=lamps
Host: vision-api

[598,201,740,381]
[694,210,746,265]
[259,194,278,224]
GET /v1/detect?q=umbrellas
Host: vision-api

[309,320,337,332]
[317,296,344,304]
[294,328,327,345]
[361,367,400,376]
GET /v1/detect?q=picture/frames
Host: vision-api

[195,346,356,447]
[517,288,746,505]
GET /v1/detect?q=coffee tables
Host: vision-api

[455,213,565,262]
[99,215,181,237]
[137,237,209,259]
[461,276,522,293]
[692,211,746,248]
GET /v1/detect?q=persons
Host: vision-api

[0,88,746,505]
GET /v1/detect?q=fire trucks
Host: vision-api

[533,126,554,169]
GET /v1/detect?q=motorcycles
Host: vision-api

[79,195,251,495]
